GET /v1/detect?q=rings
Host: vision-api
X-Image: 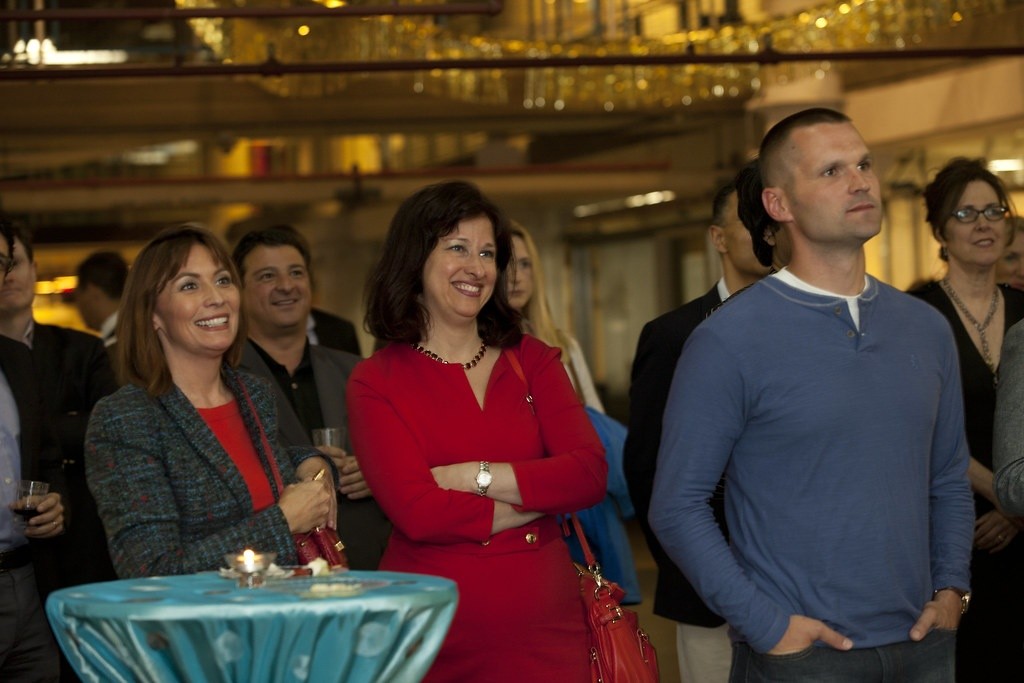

[51,520,57,528]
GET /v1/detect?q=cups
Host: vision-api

[12,480,50,534]
[311,427,347,492]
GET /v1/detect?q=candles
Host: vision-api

[238,555,264,572]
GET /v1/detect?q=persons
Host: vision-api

[342,178,609,683]
[643,107,973,682]
[1,107,1024,683]
[81,225,342,580]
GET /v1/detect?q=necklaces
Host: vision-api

[944,274,999,371]
[412,339,488,369]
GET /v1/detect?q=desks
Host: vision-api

[45,568,461,683]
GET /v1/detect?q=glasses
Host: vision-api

[952,206,1008,223]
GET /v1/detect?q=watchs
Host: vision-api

[933,586,971,614]
[477,461,493,497]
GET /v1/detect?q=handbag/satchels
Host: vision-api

[294,526,348,570]
[573,562,659,683]
